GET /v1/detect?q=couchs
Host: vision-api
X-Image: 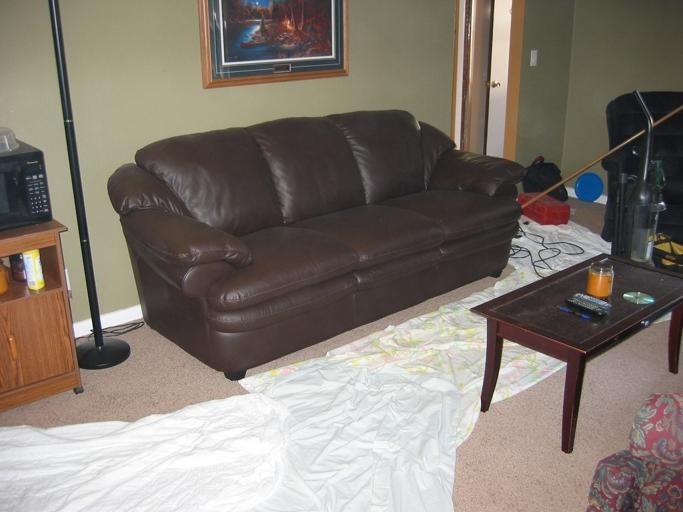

[106,108,529,382]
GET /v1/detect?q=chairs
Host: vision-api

[600,91,683,244]
[582,390,683,511]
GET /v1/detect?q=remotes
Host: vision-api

[574,292,612,309]
[566,296,607,317]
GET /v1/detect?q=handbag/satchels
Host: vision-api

[521,156,567,202]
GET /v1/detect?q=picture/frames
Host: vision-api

[197,1,351,89]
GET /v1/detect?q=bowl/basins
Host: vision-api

[0,125,21,154]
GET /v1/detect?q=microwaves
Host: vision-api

[0,141,52,232]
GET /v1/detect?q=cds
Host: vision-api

[622,291,655,305]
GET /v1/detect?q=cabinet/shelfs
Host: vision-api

[0,216,84,414]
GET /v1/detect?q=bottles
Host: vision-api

[0,248,47,304]
